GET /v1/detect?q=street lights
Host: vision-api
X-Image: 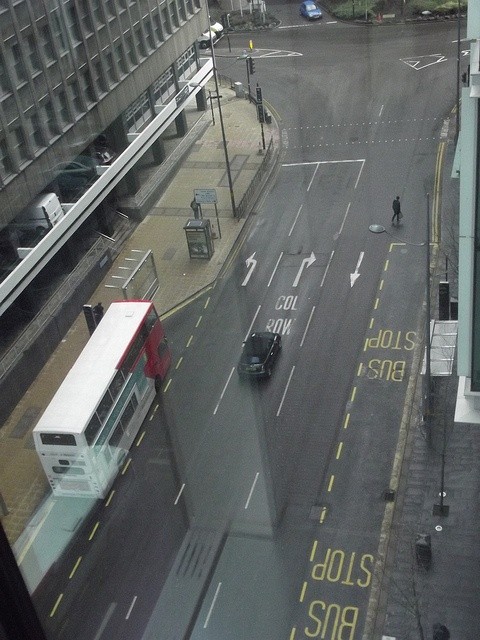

[205,0,238,220]
[190,83,214,126]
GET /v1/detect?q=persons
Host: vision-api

[92,302,104,323]
[190,197,199,219]
[391,195,403,225]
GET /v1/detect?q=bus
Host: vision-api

[32,300,174,500]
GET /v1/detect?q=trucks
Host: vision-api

[15,193,64,235]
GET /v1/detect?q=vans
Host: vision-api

[209,16,224,39]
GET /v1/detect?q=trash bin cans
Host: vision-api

[416,532,432,569]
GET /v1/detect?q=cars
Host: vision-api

[299,1,322,21]
[83,145,117,164]
[237,331,281,382]
[198,29,217,48]
[58,162,96,189]
